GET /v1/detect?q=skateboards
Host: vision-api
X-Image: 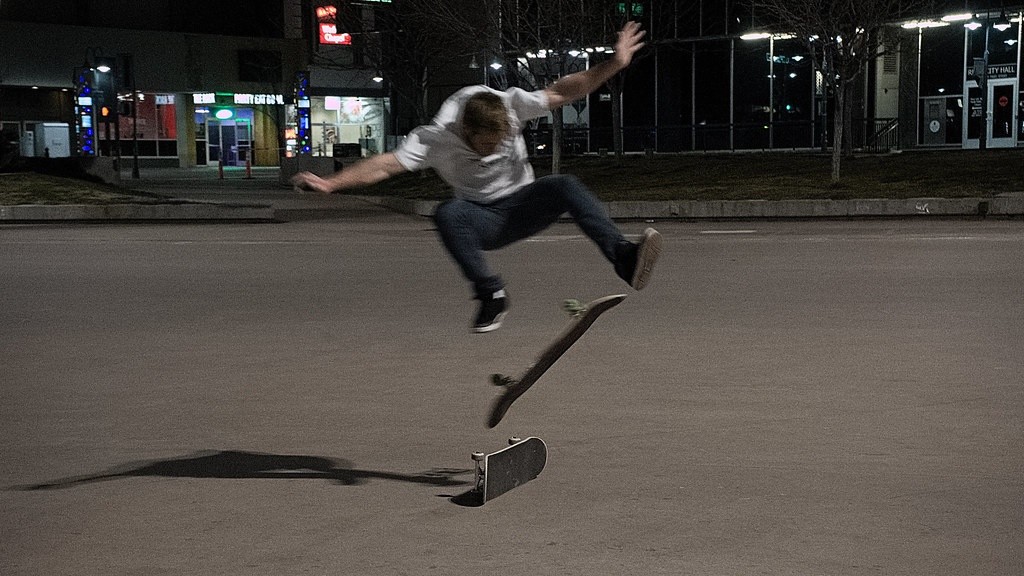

[467,436,549,504]
[479,291,631,429]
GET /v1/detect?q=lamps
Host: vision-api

[962,14,983,30]
[993,11,1012,31]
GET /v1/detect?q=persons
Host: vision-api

[291,20,664,333]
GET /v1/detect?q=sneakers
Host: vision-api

[620,226,664,292]
[469,289,511,334]
